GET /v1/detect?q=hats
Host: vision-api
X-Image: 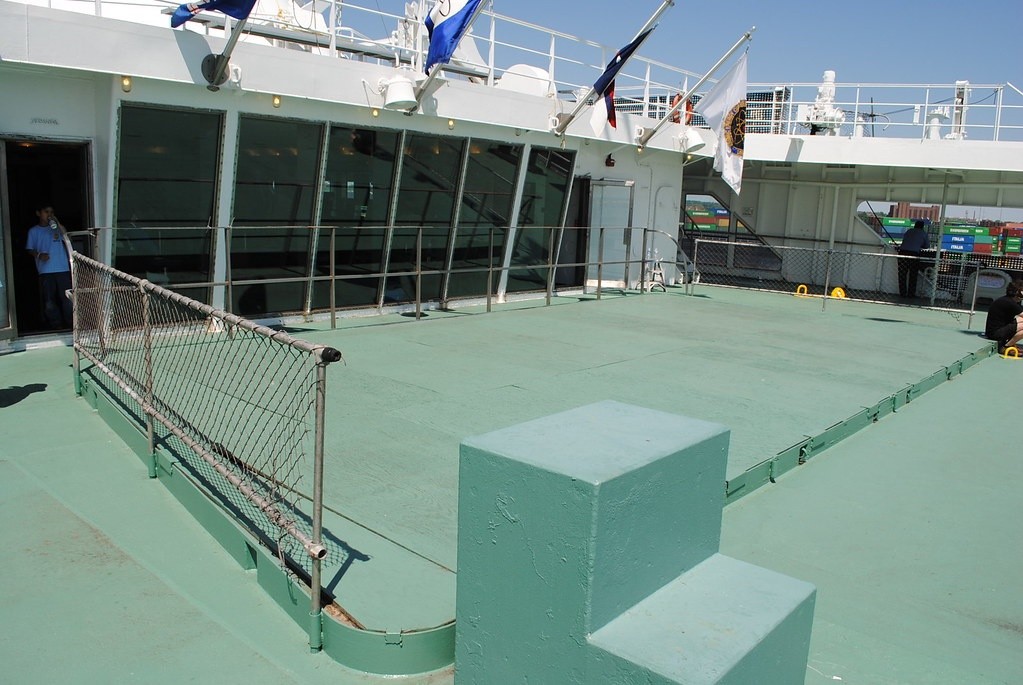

[1007,282,1023,298]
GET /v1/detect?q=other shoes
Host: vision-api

[67,323,72,328]
[1001,345,1023,356]
[56,323,64,330]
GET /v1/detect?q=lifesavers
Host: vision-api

[673,93,693,125]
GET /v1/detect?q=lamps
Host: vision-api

[585,138,590,145]
[121,76,132,92]
[448,119,456,130]
[272,95,281,108]
[514,128,521,136]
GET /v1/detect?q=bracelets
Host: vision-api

[37,252,41,258]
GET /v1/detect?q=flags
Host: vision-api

[695,53,747,195]
[171,0,257,29]
[590,27,653,137]
[425,0,481,76]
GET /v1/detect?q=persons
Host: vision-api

[898,221,930,299]
[986,280,1023,356]
[26,202,73,329]
